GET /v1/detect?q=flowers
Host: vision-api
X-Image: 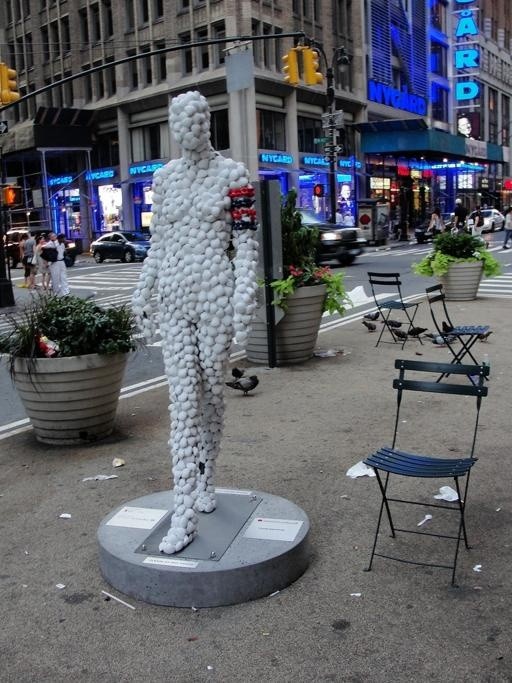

[253,183,357,317]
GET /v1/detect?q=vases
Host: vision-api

[245,280,329,366]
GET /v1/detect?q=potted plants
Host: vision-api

[1,274,150,450]
[410,228,505,302]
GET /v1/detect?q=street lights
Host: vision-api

[311,45,352,225]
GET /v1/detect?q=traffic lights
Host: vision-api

[0,62,21,104]
[4,186,23,205]
[314,184,325,196]
[304,48,324,85]
[282,48,300,88]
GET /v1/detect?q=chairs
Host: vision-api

[424,281,491,387]
[365,268,423,350]
[360,356,490,589]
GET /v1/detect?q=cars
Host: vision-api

[414,213,467,243]
[467,206,507,234]
[89,229,151,263]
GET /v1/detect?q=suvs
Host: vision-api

[3,225,77,267]
[296,208,368,267]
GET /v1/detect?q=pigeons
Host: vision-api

[361,310,457,347]
[225,374,259,397]
[231,365,244,380]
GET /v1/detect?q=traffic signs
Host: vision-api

[324,144,344,153]
[314,136,334,145]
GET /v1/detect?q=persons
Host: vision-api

[131,86,266,557]
[427,207,445,237]
[456,112,478,139]
[500,205,511,248]
[452,197,468,235]
[468,203,489,249]
[19,232,72,300]
